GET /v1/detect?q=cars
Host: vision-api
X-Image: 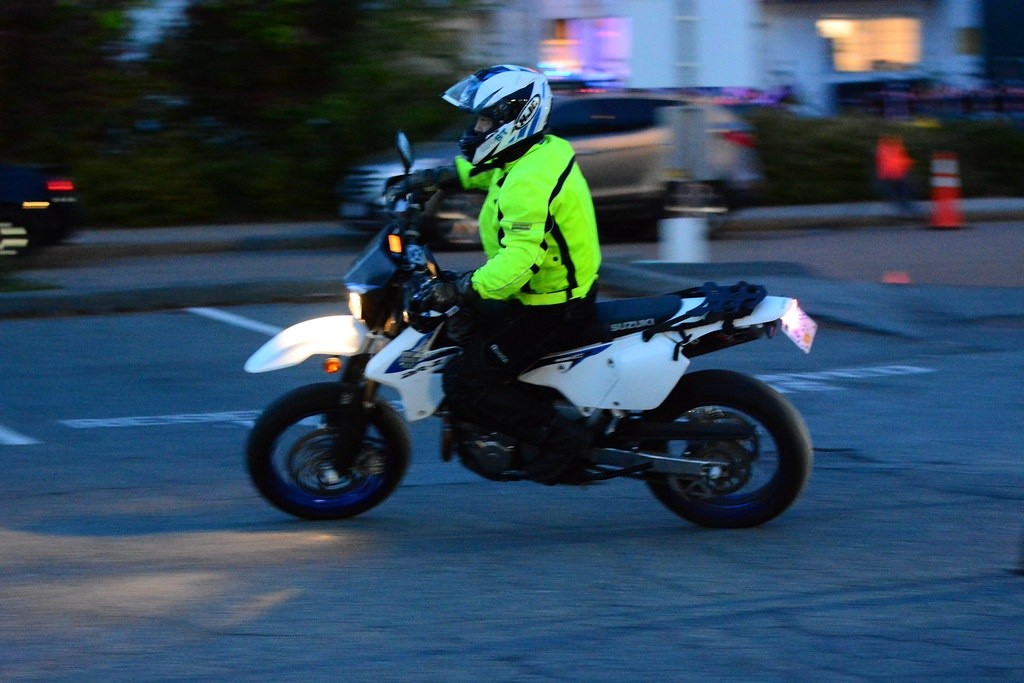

[1,161,84,269]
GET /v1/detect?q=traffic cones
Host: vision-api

[922,150,973,231]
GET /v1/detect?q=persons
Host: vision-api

[778,86,800,106]
[386,64,605,480]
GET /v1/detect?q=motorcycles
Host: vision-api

[243,129,818,528]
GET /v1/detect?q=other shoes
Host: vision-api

[535,417,595,483]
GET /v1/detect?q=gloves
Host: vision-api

[393,160,463,203]
[417,270,481,313]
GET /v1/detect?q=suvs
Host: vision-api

[335,90,768,252]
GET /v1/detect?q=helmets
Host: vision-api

[458,64,552,167]
